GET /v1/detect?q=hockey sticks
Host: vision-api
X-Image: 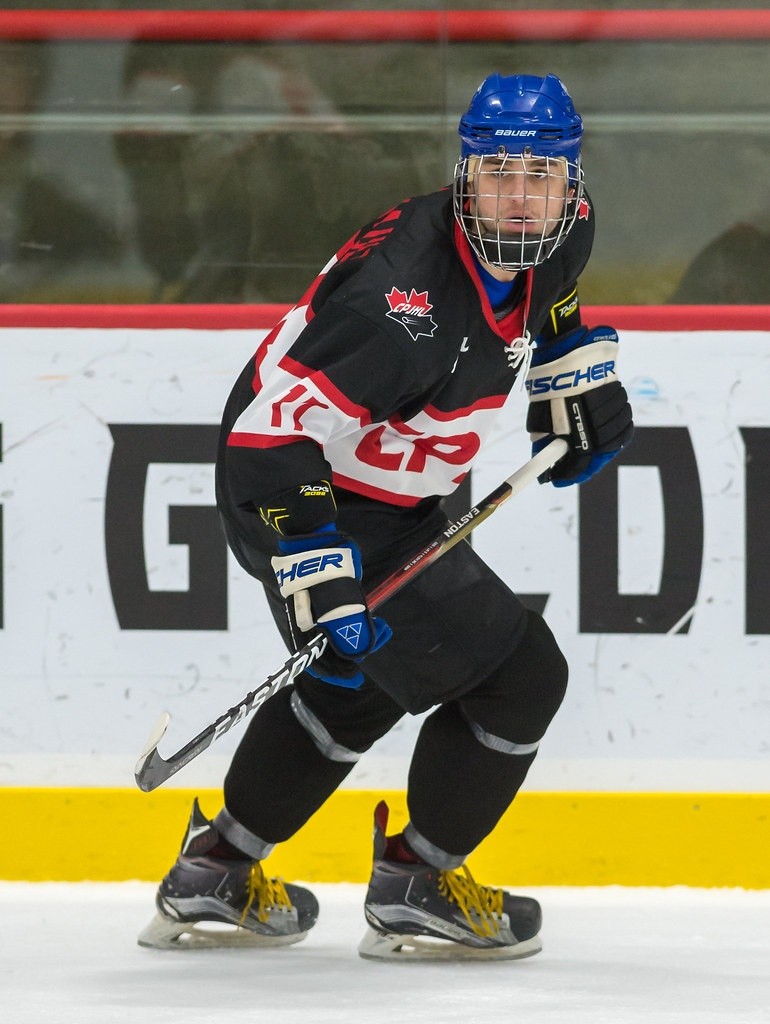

[132,432,569,796]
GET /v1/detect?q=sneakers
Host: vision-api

[136,796,319,950]
[358,801,544,961]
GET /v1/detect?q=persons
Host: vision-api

[136,71,636,964]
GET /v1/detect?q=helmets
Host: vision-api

[454,72,586,273]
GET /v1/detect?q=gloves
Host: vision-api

[271,531,394,689]
[523,325,635,487]
